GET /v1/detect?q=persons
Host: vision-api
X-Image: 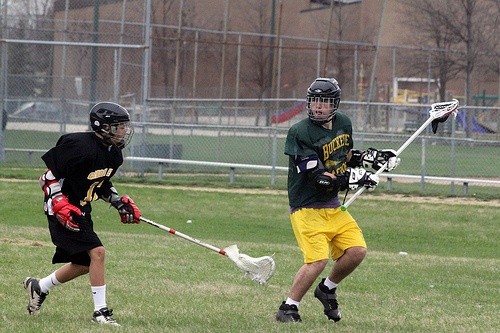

[275,77,401,326]
[23,103,141,326]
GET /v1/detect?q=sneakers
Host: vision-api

[275,301,302,323]
[314,278,341,323]
[24,277,49,317]
[93,308,121,328]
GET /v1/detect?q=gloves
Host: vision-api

[346,168,380,191]
[52,195,82,232]
[362,147,401,172]
[114,195,140,224]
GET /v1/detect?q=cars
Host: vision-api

[20,101,68,121]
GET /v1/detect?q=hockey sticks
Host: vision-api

[141,215,276,284]
[339,97,459,212]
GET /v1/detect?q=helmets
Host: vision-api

[89,102,134,152]
[306,78,341,126]
